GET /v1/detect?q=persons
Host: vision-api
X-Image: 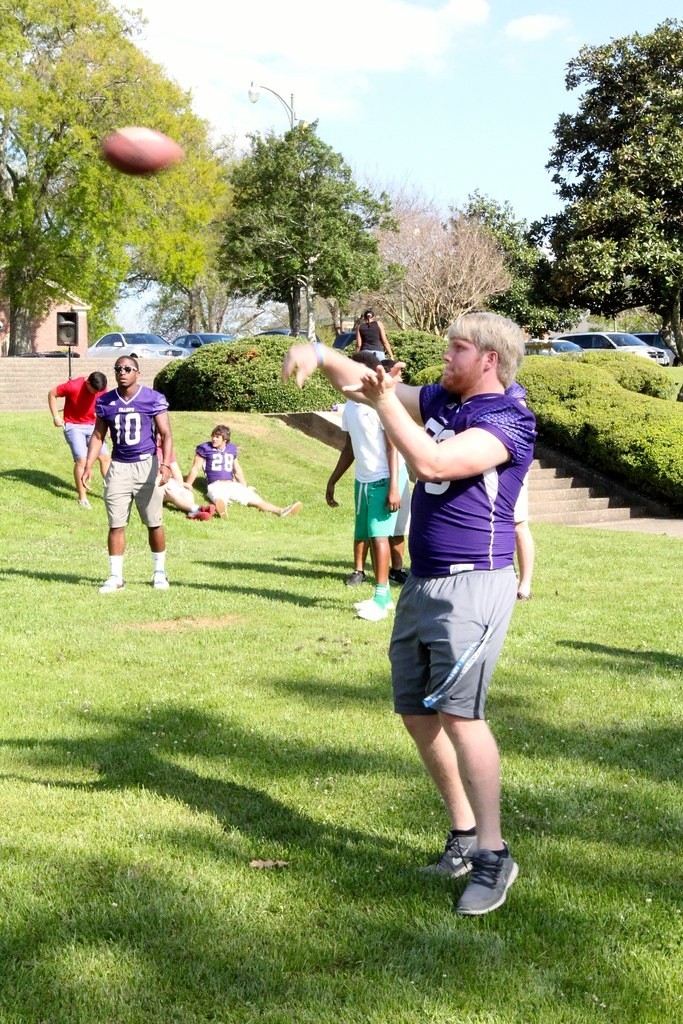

[344,360,409,585]
[156,433,215,520]
[325,350,400,621]
[47,372,109,509]
[356,311,394,361]
[185,425,301,519]
[82,356,173,593]
[504,381,534,600]
[281,311,534,915]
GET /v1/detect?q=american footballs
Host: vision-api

[102,126,184,174]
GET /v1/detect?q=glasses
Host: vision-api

[114,365,138,374]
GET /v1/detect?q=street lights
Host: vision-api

[246,79,298,336]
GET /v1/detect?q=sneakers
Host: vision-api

[345,570,366,587]
[456,840,518,914]
[388,568,409,584]
[151,570,169,589]
[420,829,478,879]
[98,574,126,594]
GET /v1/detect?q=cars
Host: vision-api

[522,339,580,355]
[169,333,256,360]
[560,331,669,368]
[629,331,682,367]
[85,332,191,360]
[259,329,321,343]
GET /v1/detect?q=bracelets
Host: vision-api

[311,341,322,366]
[159,463,170,470]
[53,416,60,419]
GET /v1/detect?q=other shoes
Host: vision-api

[216,498,228,518]
[279,501,302,517]
[353,597,395,621]
[516,591,533,600]
[77,499,93,509]
[187,504,215,520]
